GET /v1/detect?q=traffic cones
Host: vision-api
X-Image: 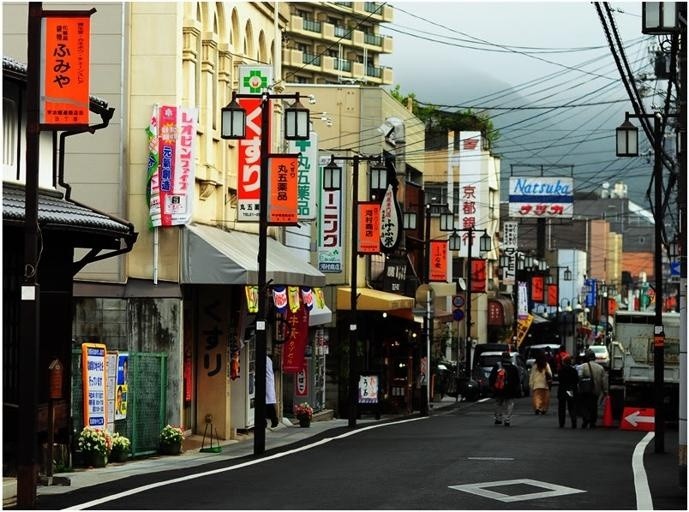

[604,396,615,427]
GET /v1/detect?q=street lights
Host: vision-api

[580,278,616,337]
[498,249,573,353]
[404,204,453,415]
[616,111,677,455]
[221,92,309,454]
[449,228,490,404]
[323,155,387,427]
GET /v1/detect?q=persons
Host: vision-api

[529,343,609,428]
[489,352,522,427]
[265,355,279,427]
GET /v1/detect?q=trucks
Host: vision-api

[610,308,679,419]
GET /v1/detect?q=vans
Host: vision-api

[527,344,561,370]
[473,344,529,397]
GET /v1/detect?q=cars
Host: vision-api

[588,344,610,367]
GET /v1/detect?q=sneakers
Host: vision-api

[495,421,510,427]
[535,410,546,415]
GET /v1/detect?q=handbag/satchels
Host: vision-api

[493,367,508,392]
[577,378,594,394]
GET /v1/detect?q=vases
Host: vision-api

[297,415,311,428]
[114,447,128,463]
[85,445,109,466]
[163,438,182,455]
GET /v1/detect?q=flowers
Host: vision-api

[292,402,314,421]
[157,423,185,443]
[111,431,131,451]
[77,426,115,452]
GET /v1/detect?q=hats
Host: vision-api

[501,352,513,359]
